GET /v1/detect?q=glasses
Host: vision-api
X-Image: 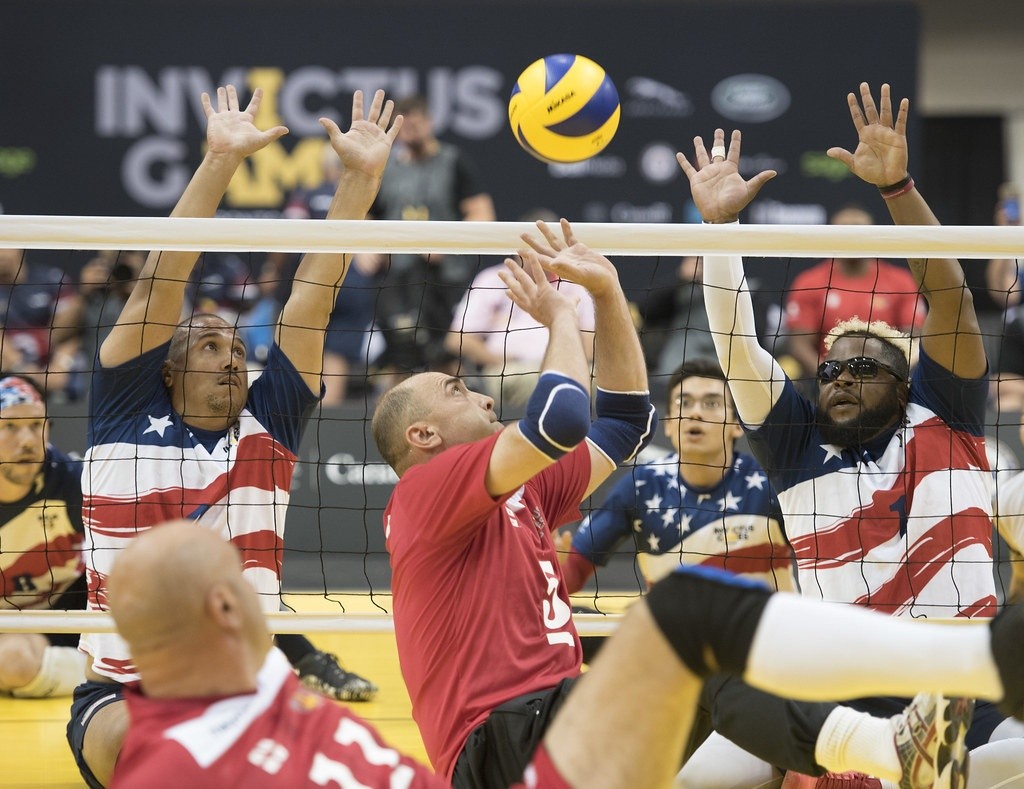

[815,357,904,385]
[676,395,734,413]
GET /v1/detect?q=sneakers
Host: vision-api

[290,651,379,701]
[890,691,976,789]
[989,584,1024,724]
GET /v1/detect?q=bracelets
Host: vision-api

[877,173,914,200]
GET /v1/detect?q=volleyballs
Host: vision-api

[506,51,622,164]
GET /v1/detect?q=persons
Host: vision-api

[0,81,1024,789]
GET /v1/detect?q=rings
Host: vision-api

[711,147,725,163]
